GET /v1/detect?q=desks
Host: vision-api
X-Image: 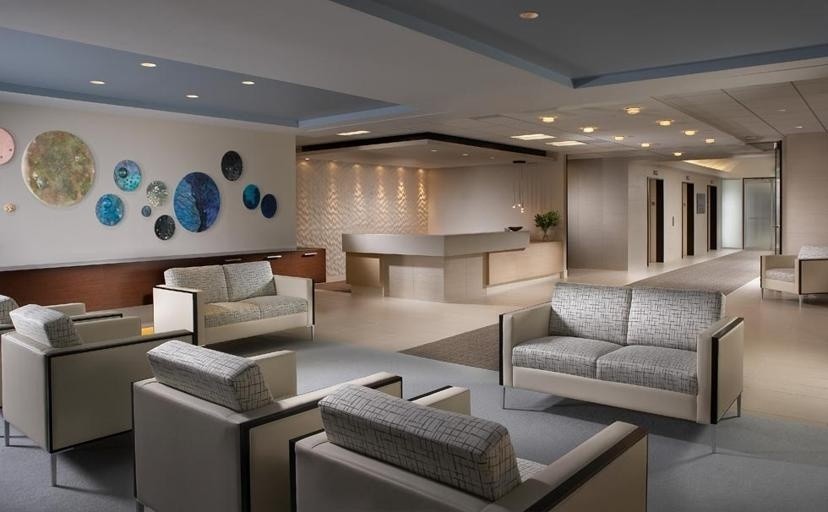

[0,248,326,312]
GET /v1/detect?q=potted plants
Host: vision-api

[532,210,562,241]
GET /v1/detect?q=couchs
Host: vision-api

[131,338,403,510]
[153,260,317,349]
[498,282,745,452]
[1,293,194,488]
[288,385,649,512]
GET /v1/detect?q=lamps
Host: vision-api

[512,160,528,216]
[536,106,716,158]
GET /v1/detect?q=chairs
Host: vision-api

[759,246,828,312]
